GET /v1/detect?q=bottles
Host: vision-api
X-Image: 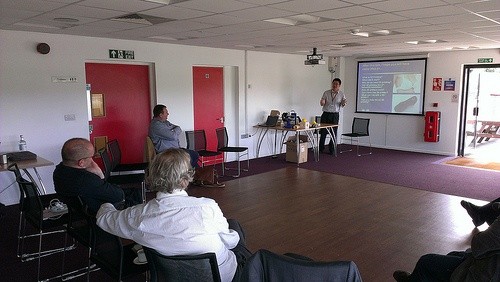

[0,152,7,164]
[18,135,27,152]
[305,120,309,130]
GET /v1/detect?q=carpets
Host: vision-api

[0,143,500,282]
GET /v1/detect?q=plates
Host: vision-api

[133,257,148,264]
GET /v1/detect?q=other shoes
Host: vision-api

[393,270,412,282]
[461,200,485,227]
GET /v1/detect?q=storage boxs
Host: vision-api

[282,134,308,163]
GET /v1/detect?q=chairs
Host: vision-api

[0,115,373,282]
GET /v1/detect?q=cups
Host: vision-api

[137,249,147,262]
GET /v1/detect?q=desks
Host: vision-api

[0,150,55,198]
[254,123,340,163]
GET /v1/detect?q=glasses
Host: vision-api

[79,153,97,161]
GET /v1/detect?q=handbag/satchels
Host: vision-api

[43,198,71,232]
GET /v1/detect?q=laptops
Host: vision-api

[258,115,279,127]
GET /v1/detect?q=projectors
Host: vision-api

[304,59,327,66]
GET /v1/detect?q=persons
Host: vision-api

[96,148,253,282]
[148,105,200,167]
[53,138,138,213]
[393,198,500,282]
[319,78,347,154]
